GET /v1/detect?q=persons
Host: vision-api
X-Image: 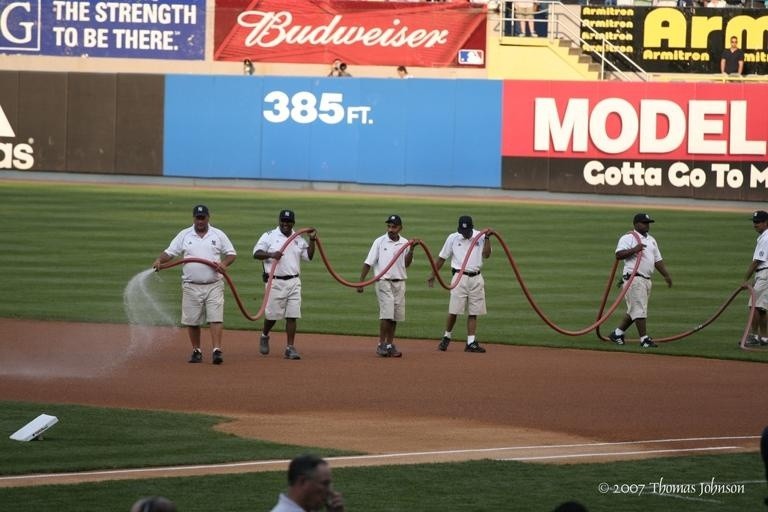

[739,211,768,345]
[562,0,753,8]
[609,213,672,348]
[397,66,412,79]
[270,454,345,512]
[329,58,352,77]
[252,209,316,360]
[358,215,419,357]
[153,204,237,364]
[515,1,538,37]
[720,36,745,74]
[426,216,493,352]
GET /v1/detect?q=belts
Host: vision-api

[755,267,768,271]
[386,279,400,281]
[629,273,650,279]
[455,270,480,277]
[273,274,299,279]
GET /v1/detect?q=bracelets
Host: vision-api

[309,238,316,241]
[409,250,413,254]
[485,237,489,240]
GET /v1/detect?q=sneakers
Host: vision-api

[189,349,202,362]
[259,335,269,354]
[284,346,300,359]
[464,341,485,352]
[376,343,402,357]
[608,331,625,344]
[438,336,450,350]
[213,350,223,364]
[738,337,760,345]
[641,337,658,347]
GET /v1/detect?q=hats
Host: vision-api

[385,215,401,224]
[194,205,208,217]
[279,210,294,220]
[633,214,654,223]
[458,216,470,233]
[749,211,768,221]
